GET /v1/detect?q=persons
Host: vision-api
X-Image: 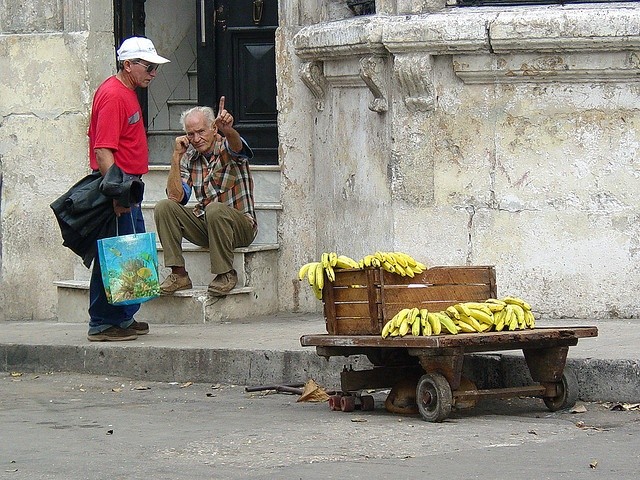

[153,95,258,296]
[87,38,171,342]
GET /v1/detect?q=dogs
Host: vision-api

[385,370,481,416]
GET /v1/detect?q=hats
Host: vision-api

[117,37,171,64]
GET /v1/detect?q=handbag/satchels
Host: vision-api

[97,210,160,305]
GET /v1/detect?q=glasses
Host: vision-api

[131,61,160,73]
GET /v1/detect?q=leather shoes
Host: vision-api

[87,324,138,341]
[160,271,192,296]
[124,319,150,335]
[207,269,239,295]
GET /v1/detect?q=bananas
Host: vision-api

[441,302,505,333]
[485,298,536,332]
[359,252,427,278]
[382,307,457,339]
[299,251,359,300]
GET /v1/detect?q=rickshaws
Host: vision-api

[299,263,598,424]
